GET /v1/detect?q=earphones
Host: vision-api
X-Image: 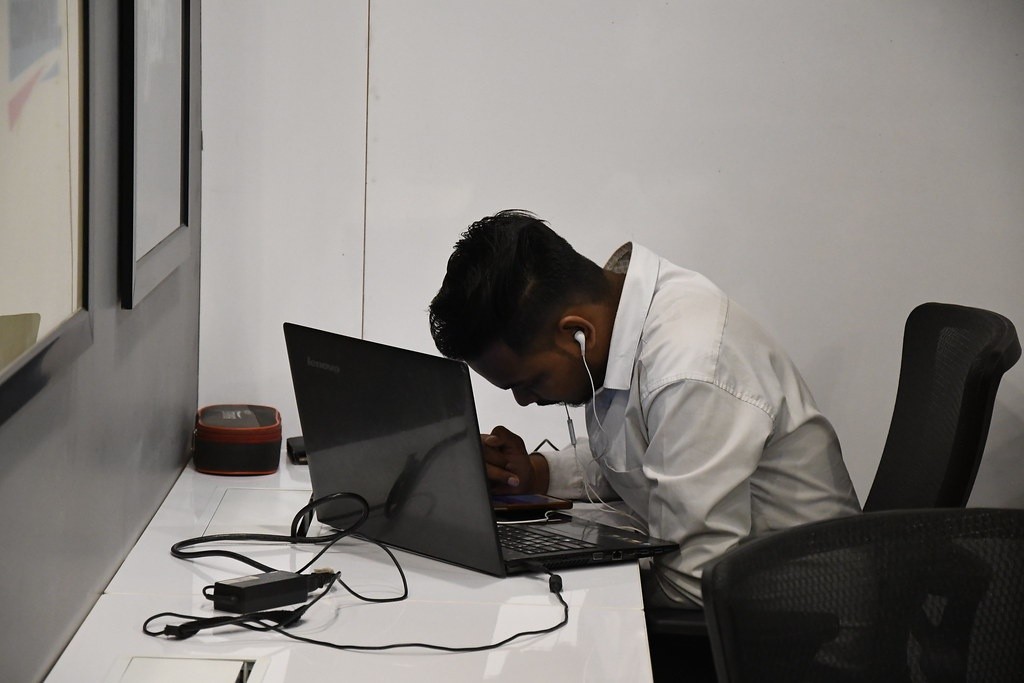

[574,330,586,358]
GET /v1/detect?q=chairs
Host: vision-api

[699,507,1024,683]
[645,299,1022,642]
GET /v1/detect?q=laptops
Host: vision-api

[284,323,679,580]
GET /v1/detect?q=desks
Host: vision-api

[38,446,654,683]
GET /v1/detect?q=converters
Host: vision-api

[214,570,308,615]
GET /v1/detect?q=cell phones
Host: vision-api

[493,492,572,517]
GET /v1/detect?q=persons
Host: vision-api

[429,209,879,683]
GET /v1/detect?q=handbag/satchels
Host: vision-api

[190,404,286,476]
